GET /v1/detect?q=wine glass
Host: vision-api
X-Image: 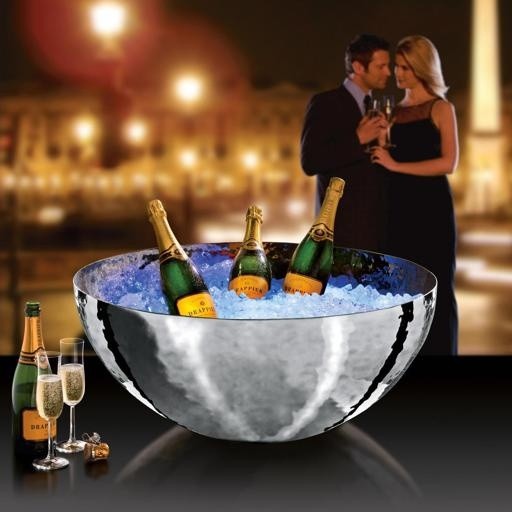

[364,94,398,155]
[33,349,70,469]
[58,337,90,452]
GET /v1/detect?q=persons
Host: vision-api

[363,34,459,357]
[296,29,397,253]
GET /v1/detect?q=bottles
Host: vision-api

[147,199,220,316]
[283,176,345,298]
[229,206,272,298]
[13,302,58,475]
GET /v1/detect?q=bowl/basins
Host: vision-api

[72,242,437,444]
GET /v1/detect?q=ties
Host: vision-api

[364,95,371,116]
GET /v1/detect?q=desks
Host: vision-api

[1,350,512,512]
